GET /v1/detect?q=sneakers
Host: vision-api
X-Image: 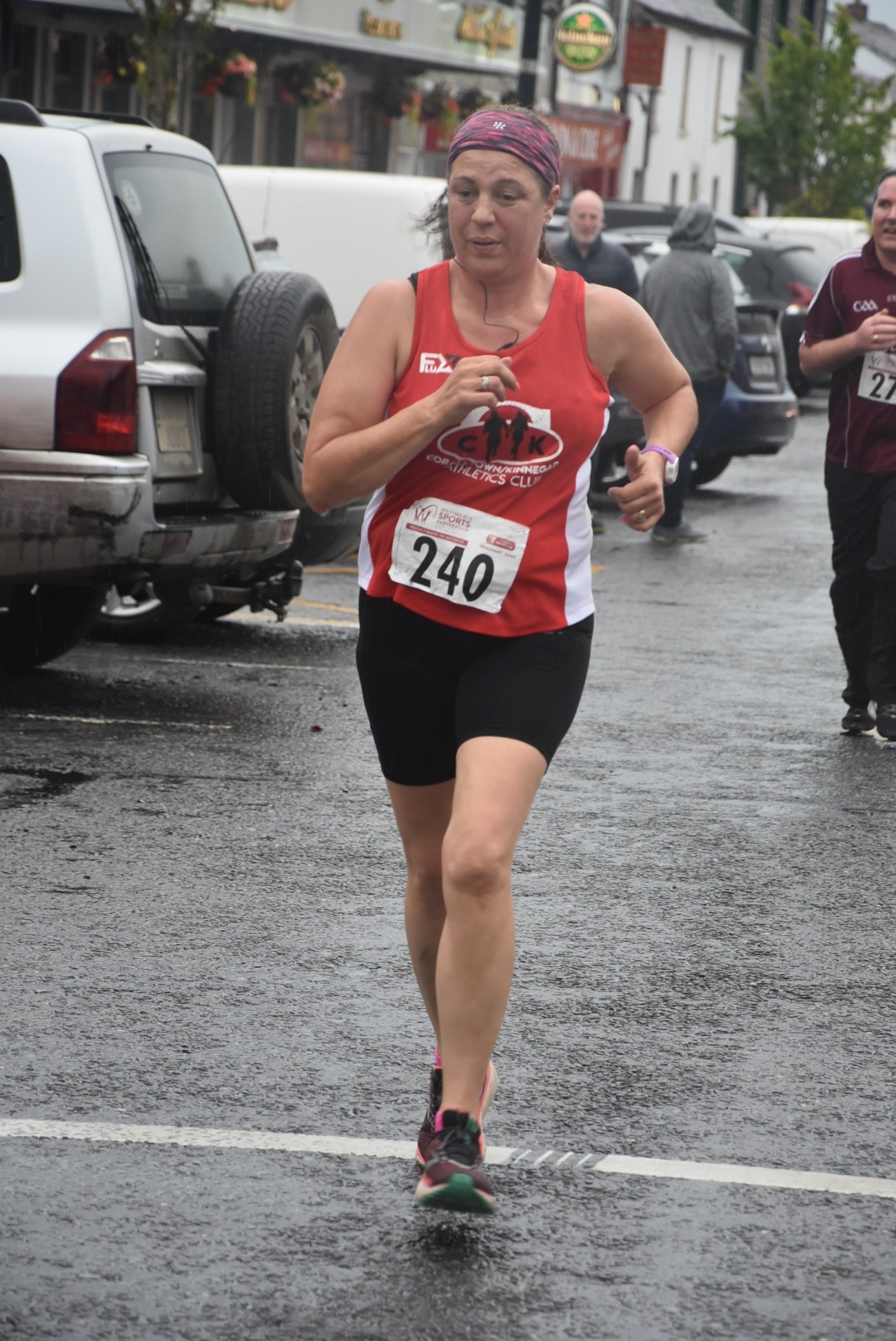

[841,702,876,730]
[591,515,604,532]
[651,524,705,540]
[876,705,896,737]
[416,1048,497,1169]
[413,1109,496,1213]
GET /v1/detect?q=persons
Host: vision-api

[302,104,700,1227]
[640,201,740,544]
[796,168,896,738]
[548,187,640,532]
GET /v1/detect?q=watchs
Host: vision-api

[639,444,679,486]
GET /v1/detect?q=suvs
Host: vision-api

[208,161,873,504]
[0,91,339,674]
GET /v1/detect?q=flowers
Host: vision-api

[199,48,259,104]
[369,76,495,129]
[274,61,345,111]
[96,29,149,88]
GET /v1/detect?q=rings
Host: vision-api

[482,376,490,390]
[873,334,878,340]
[638,509,645,523]
